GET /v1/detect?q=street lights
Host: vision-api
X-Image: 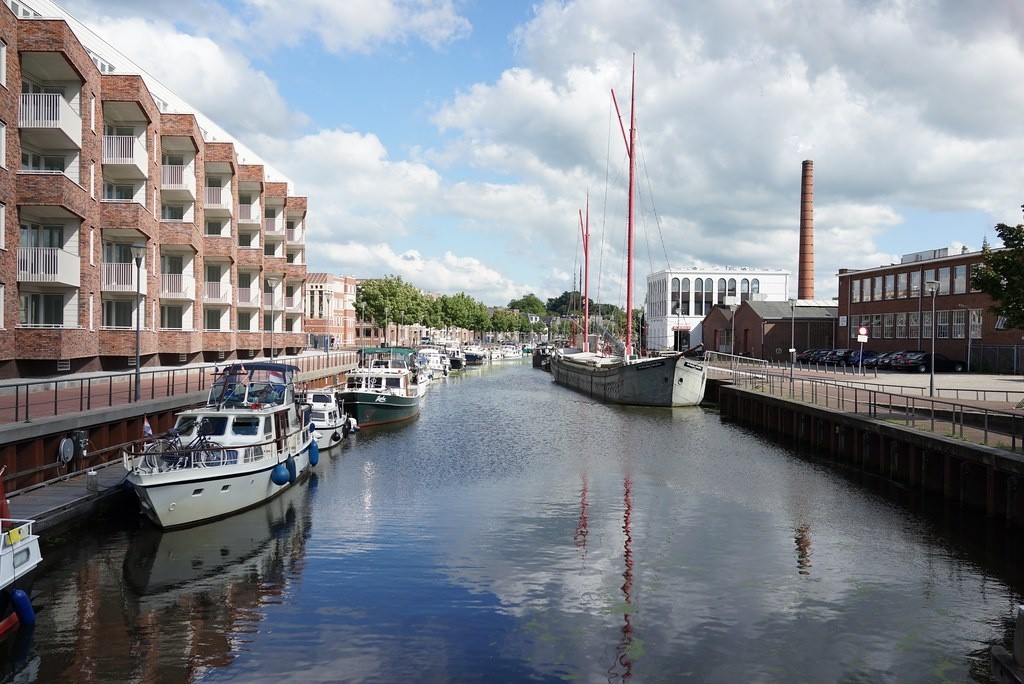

[958,304,971,373]
[268,277,280,362]
[925,281,940,397]
[676,308,681,352]
[130,245,148,401]
[788,299,797,381]
[638,312,644,359]
[730,304,738,370]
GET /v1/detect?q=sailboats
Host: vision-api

[549,50,707,408]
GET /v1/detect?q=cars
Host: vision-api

[797,349,967,374]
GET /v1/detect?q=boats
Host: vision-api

[338,347,421,427]
[120,362,319,532]
[533,341,556,373]
[415,341,537,397]
[0,465,44,662]
[294,388,360,451]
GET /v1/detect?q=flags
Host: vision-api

[142,413,153,438]
